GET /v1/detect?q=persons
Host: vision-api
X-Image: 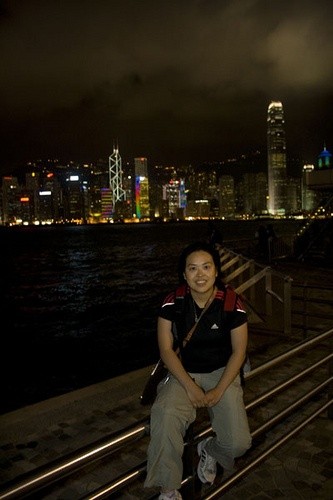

[144,242,252,500]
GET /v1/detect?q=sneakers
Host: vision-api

[197,436,217,485]
[158,489,182,500]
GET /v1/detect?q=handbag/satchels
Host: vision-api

[140,359,168,406]
